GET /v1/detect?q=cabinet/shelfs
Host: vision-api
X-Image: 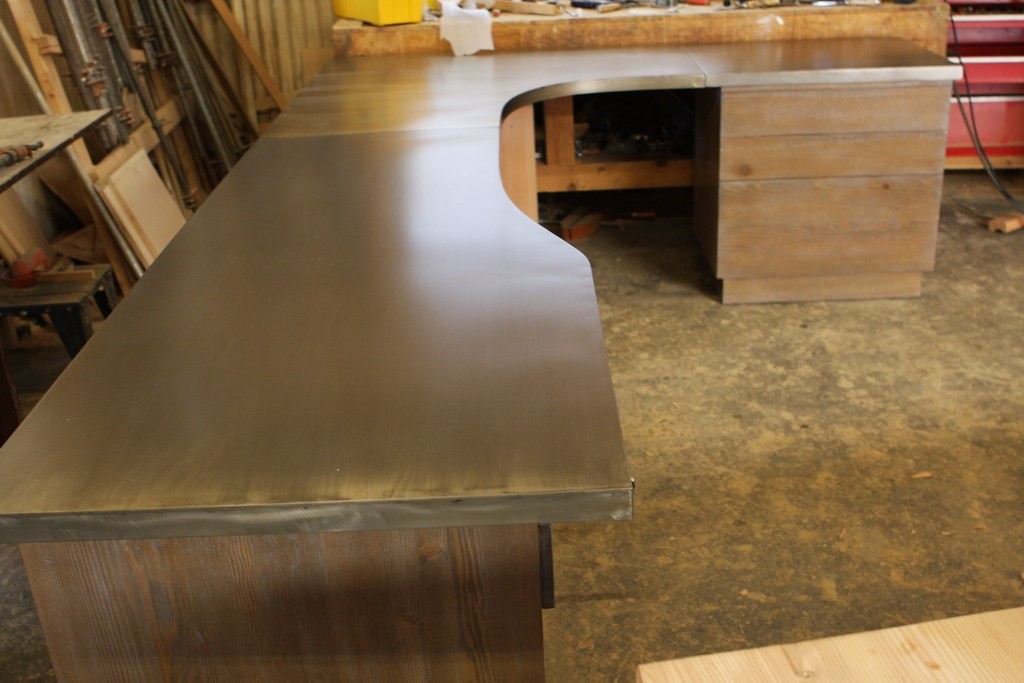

[695,77,955,305]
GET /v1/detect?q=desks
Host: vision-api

[2,38,964,683]
[331,1,952,59]
